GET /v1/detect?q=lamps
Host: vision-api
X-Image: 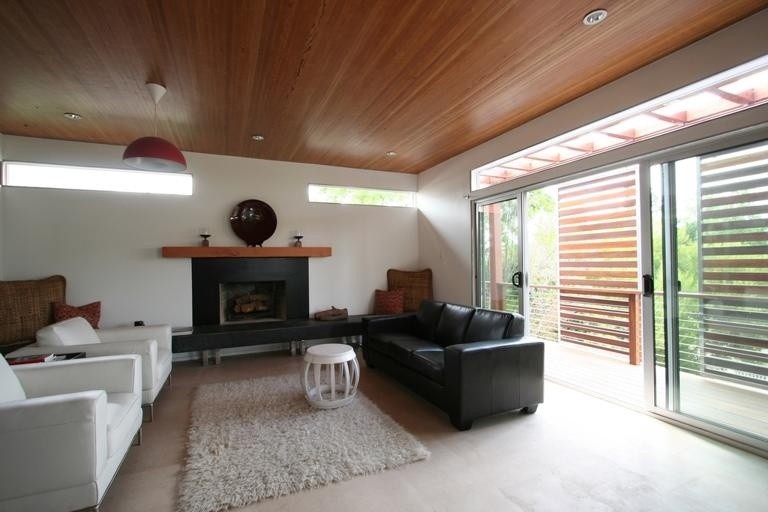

[64,113,82,121]
[122,78,188,172]
[582,9,607,26]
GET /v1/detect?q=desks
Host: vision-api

[172,312,372,366]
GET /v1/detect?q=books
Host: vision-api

[6,353,57,365]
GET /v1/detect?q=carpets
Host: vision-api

[176,369,430,511]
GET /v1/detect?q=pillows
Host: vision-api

[430,302,476,348]
[374,288,404,315]
[0,275,66,347]
[465,308,524,347]
[387,268,432,313]
[51,301,101,329]
[412,300,446,340]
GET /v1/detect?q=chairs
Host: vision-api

[35,316,173,423]
[0,354,144,511]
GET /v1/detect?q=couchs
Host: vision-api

[361,299,544,432]
